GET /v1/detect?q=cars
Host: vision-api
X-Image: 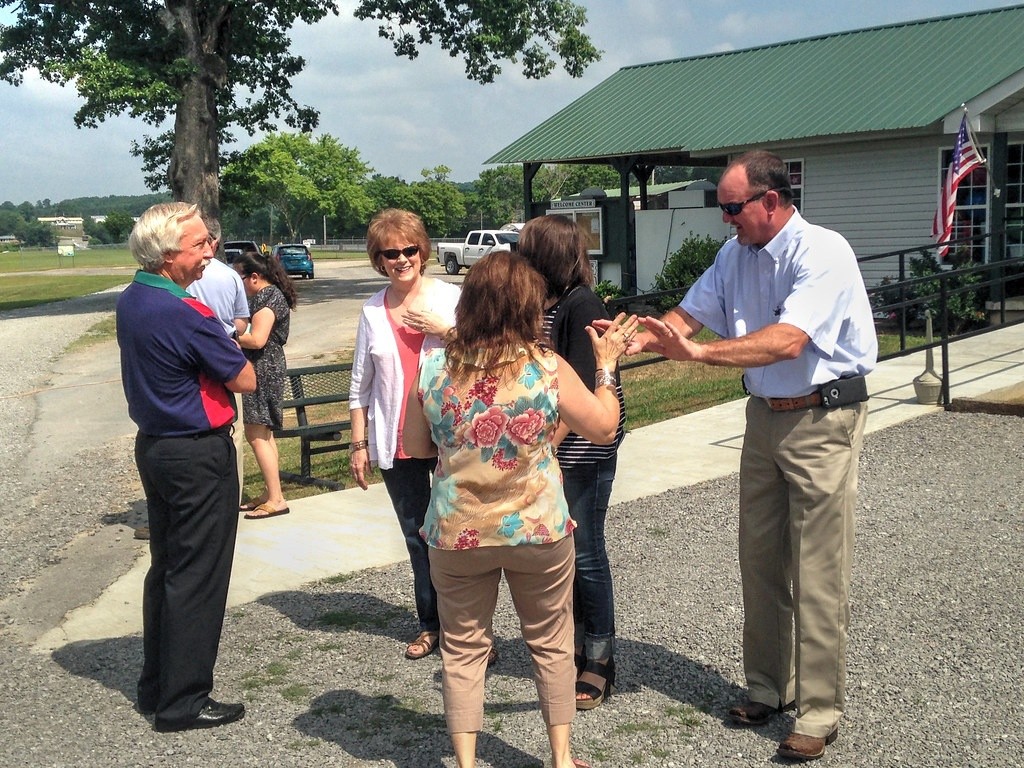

[270,244,314,280]
[224,241,262,254]
[225,249,243,268]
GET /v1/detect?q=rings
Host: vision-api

[623,332,628,337]
[413,323,418,328]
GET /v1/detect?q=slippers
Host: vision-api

[244,504,289,519]
[240,496,267,511]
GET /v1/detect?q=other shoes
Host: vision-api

[573,759,590,768]
[728,699,796,724]
[134,527,150,539]
[776,728,837,759]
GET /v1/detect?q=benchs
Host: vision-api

[272,362,371,491]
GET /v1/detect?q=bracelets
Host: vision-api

[445,327,456,341]
[595,369,616,389]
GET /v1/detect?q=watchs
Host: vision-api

[349,440,366,455]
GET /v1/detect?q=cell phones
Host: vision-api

[820,376,868,408]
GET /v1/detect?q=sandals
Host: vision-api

[574,644,586,679]
[488,639,498,666]
[405,630,440,660]
[575,656,615,709]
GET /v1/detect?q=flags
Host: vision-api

[931,114,984,257]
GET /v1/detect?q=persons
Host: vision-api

[422,249,639,768]
[181,215,290,520]
[596,150,878,759]
[516,213,629,710]
[349,210,496,666]
[116,202,258,732]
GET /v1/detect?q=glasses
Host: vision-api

[210,234,220,252]
[719,185,780,215]
[379,244,420,259]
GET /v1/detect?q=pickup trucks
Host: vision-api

[436,230,520,275]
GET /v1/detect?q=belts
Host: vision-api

[771,392,821,411]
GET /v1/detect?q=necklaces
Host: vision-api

[393,277,423,313]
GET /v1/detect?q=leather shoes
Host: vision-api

[136,697,246,732]
